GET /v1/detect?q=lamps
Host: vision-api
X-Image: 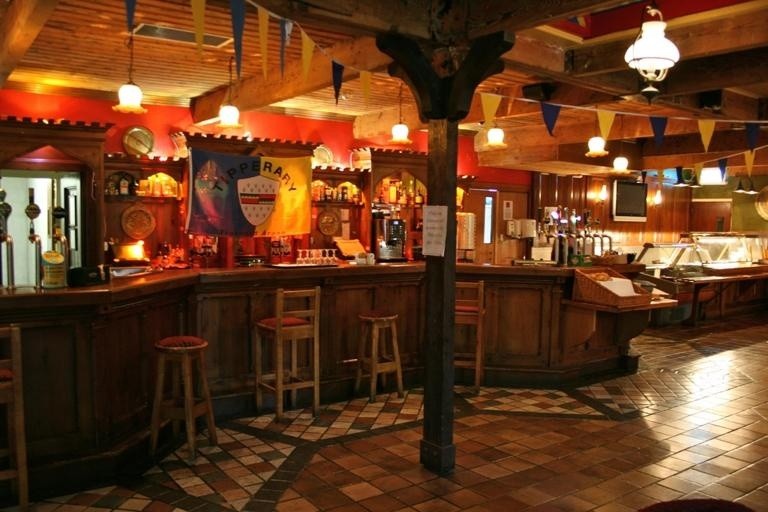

[746,176,759,194]
[487,119,504,146]
[113,27,147,113]
[624,0,680,94]
[585,102,609,158]
[392,83,409,144]
[733,176,745,194]
[613,114,628,172]
[218,56,239,126]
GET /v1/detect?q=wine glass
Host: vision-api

[294,247,339,267]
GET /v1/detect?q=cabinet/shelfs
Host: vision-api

[638,230,768,340]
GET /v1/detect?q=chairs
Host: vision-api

[255,286,321,418]
[0,322,30,512]
[453,279,488,394]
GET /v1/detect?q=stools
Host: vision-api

[352,311,405,402]
[149,335,218,460]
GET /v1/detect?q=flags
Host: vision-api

[184,146,312,238]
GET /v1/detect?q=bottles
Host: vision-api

[312,185,362,206]
[103,173,164,198]
[372,175,425,207]
[531,204,582,264]
[154,238,183,271]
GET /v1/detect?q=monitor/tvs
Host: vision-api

[612,179,647,223]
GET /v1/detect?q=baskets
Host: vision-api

[572,267,651,306]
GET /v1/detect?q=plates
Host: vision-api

[313,146,334,169]
[316,211,340,236]
[120,206,156,238]
[120,125,155,157]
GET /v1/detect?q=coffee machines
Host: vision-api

[370,202,410,261]
